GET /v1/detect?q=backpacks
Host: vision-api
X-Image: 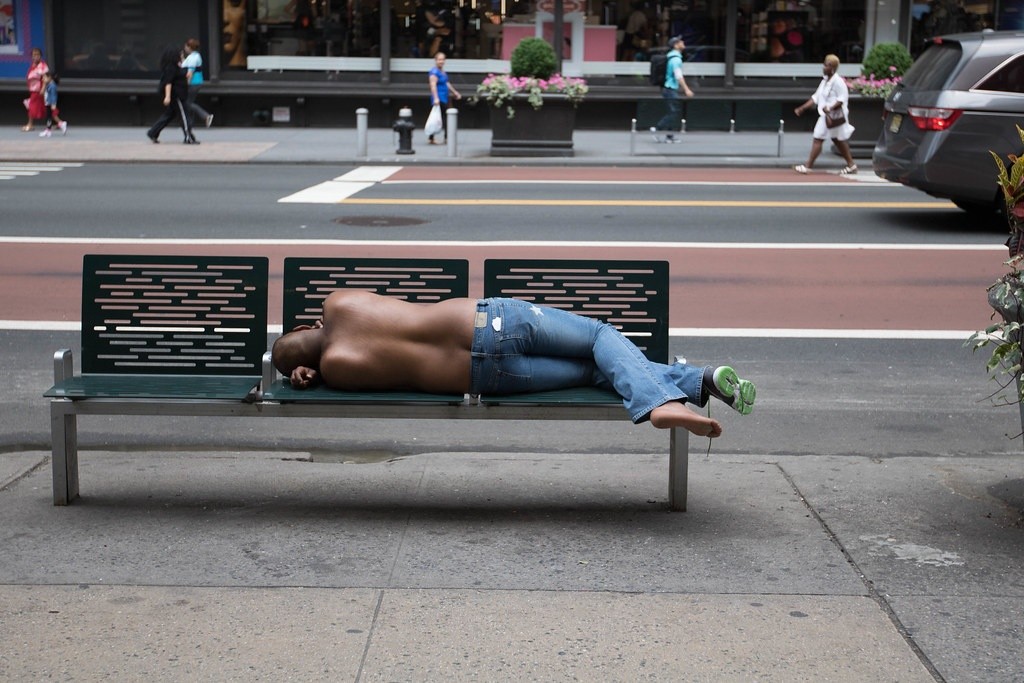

[650,54,681,87]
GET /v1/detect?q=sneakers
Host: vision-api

[703,366,756,414]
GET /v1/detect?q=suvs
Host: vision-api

[872,29,1024,230]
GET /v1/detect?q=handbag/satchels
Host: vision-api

[424,104,442,136]
[826,107,846,129]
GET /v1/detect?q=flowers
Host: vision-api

[468,36,588,120]
[841,40,913,99]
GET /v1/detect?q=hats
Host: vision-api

[668,35,682,47]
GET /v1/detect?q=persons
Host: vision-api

[426,52,461,145]
[271,289,756,438]
[649,35,695,143]
[38,70,68,137]
[292,0,476,59]
[85,42,140,72]
[180,38,213,128]
[222,0,247,67]
[21,48,50,132]
[621,0,651,61]
[793,54,859,174]
[147,46,201,144]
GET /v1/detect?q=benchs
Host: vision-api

[43,255,690,512]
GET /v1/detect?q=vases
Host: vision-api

[829,92,885,158]
[468,93,585,157]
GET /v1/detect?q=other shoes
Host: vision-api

[21,125,35,132]
[38,130,51,137]
[649,127,660,143]
[840,163,857,174]
[60,121,67,136]
[793,165,816,175]
[665,135,681,144]
[206,114,213,128]
[185,134,200,144]
[147,129,160,143]
